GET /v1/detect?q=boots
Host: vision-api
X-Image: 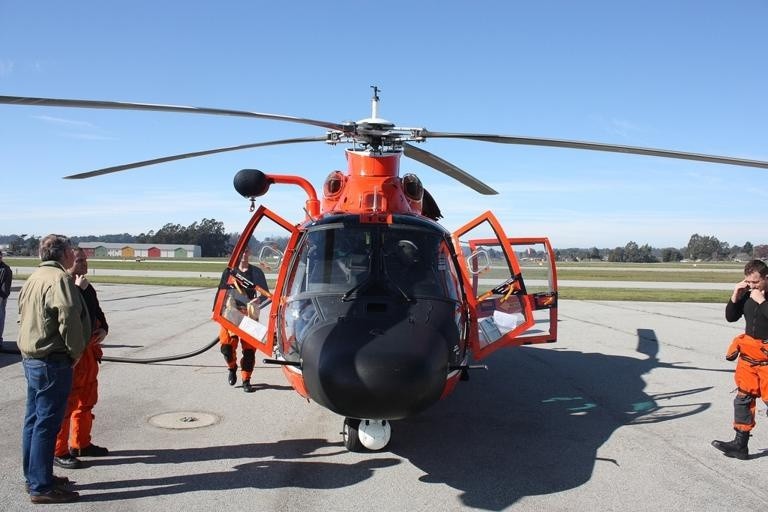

[711,429,750,460]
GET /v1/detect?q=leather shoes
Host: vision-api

[70,444,108,457]
[243,379,252,392]
[53,453,82,469]
[24,476,80,503]
[228,364,238,385]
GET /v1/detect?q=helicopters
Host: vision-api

[0,85,768,453]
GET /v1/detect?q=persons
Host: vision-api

[711,260,768,460]
[219,244,269,392]
[0,251,12,343]
[17,234,108,504]
